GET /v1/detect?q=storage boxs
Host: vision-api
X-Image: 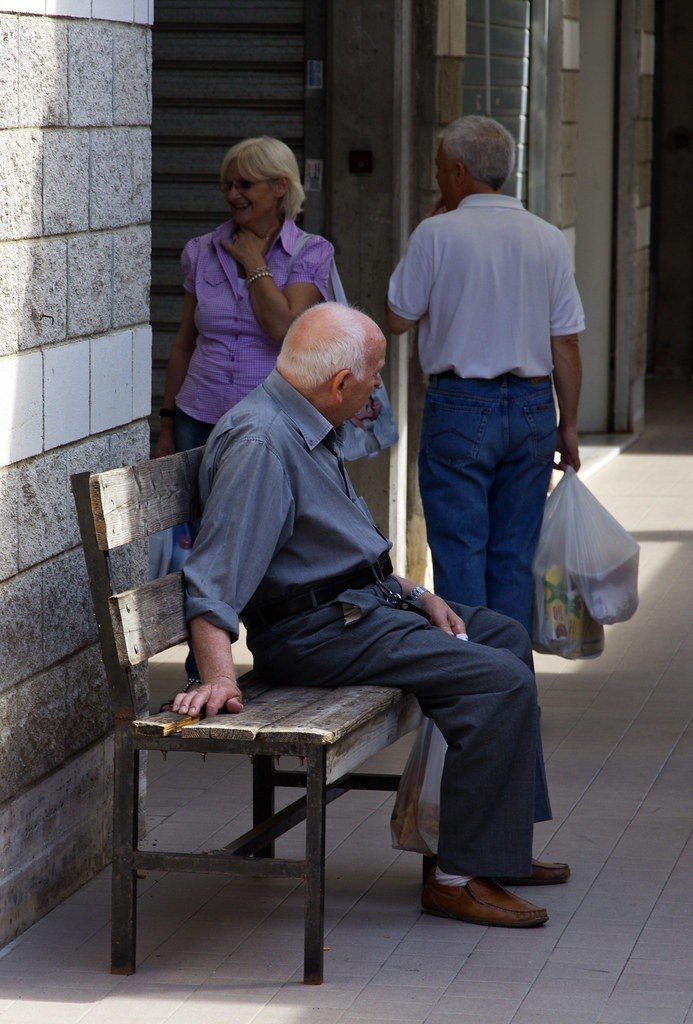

[544,564,604,658]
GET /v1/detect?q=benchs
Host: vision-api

[69,445,434,986]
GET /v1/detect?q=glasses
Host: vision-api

[218,178,272,191]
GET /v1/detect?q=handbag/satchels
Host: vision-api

[532,464,639,659]
[290,230,399,461]
[390,709,449,854]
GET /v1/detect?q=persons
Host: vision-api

[150,136,334,712]
[174,302,570,927]
[384,115,584,632]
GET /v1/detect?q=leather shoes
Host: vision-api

[499,858,570,884]
[421,867,550,928]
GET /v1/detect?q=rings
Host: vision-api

[233,237,238,244]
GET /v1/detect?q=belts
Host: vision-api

[246,554,393,634]
[436,370,550,386]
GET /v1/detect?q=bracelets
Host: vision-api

[159,409,175,418]
[247,266,269,280]
[247,273,273,287]
[406,586,429,601]
